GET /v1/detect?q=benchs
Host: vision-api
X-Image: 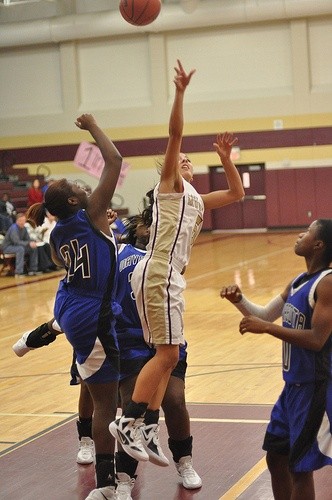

[0,161,128,274]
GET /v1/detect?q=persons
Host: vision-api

[108,60,245,467]
[220,217,332,500]
[0,114,204,500]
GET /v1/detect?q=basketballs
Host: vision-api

[118,0,162,27]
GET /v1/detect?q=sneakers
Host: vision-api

[76,434,96,464]
[15,264,60,279]
[172,455,202,489]
[109,415,150,462]
[136,422,170,466]
[85,472,137,500]
[12,324,41,357]
